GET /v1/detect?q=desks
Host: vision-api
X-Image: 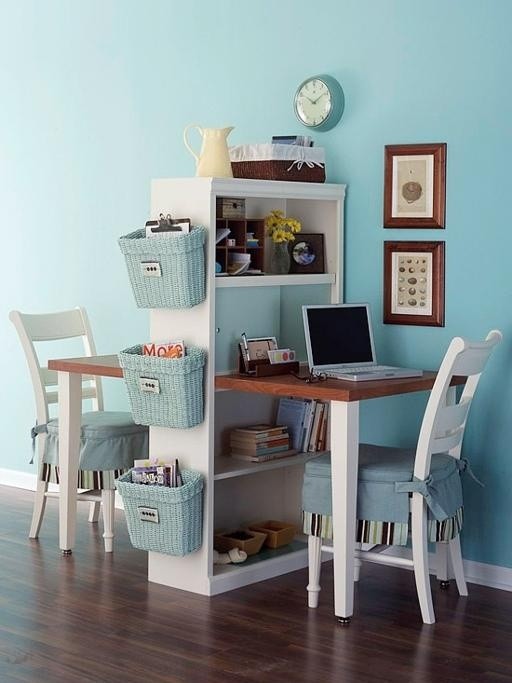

[47,351,124,554]
[214,363,476,622]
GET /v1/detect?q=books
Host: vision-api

[271,134,314,146]
[162,347,181,358]
[130,454,185,489]
[228,260,250,275]
[246,336,278,349]
[238,340,275,372]
[227,251,251,274]
[215,227,232,244]
[141,339,185,358]
[230,397,327,463]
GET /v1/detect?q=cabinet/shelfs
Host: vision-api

[145,176,347,597]
[215,217,265,278]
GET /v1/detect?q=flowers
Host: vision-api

[266,211,300,243]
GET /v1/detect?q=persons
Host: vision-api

[295,244,315,264]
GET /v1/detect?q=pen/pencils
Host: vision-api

[242,332,251,362]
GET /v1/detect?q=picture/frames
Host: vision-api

[287,232,325,274]
[385,142,446,228]
[385,240,446,327]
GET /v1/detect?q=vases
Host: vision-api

[271,236,287,273]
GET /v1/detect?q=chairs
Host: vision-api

[302,329,501,624]
[9,306,150,552]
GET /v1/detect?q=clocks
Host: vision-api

[294,72,344,130]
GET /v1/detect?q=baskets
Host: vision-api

[114,468,204,557]
[117,345,206,428]
[230,160,326,182]
[250,520,297,548]
[216,528,267,555]
[119,226,206,308]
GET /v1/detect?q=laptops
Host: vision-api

[299,302,423,382]
